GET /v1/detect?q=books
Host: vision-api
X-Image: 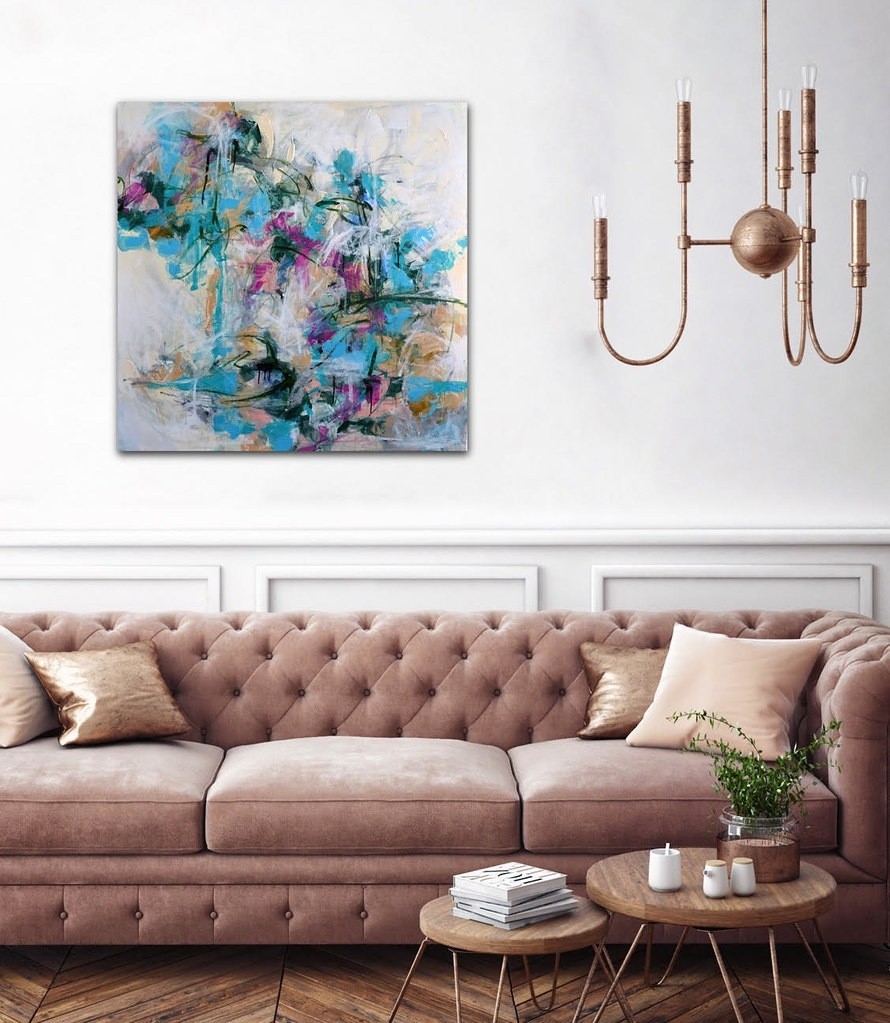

[453,908,575,930]
[458,898,580,923]
[449,888,561,907]
[456,889,573,915]
[449,862,568,901]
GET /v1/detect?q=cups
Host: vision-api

[702,859,728,899]
[647,848,682,893]
[730,857,756,896]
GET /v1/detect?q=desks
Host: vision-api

[586,846,849,1023]
[386,893,639,1023]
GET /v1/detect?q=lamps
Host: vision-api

[591,0,872,367]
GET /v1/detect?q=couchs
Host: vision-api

[0,610,889,947]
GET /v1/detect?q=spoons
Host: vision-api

[665,842,671,856]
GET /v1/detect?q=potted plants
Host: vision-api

[665,709,844,882]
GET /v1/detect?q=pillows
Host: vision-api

[23,639,191,747]
[578,642,669,739]
[0,624,60,748]
[626,621,823,761]
